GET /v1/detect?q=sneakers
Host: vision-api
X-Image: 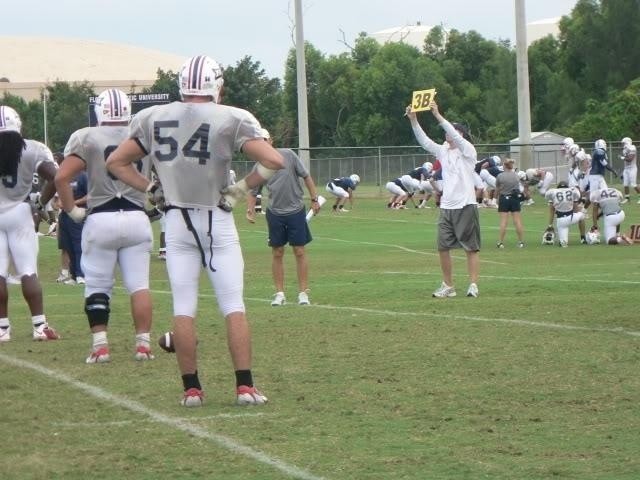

[0,325,11,341]
[559,235,633,248]
[135,343,155,360]
[86,347,110,363]
[271,292,286,306]
[237,386,268,405]
[298,292,309,305]
[332,208,348,212]
[497,242,504,248]
[181,388,203,406]
[517,242,525,248]
[467,283,478,297]
[478,198,498,208]
[387,202,430,209]
[159,248,166,259]
[432,281,456,297]
[56,273,85,285]
[37,222,56,237]
[33,323,60,340]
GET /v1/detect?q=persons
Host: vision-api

[384,134,618,212]
[494,156,527,251]
[543,181,587,249]
[617,135,640,203]
[326,171,361,215]
[18,135,268,286]
[589,186,635,246]
[54,84,153,364]
[103,55,284,408]
[0,101,61,346]
[246,128,323,309]
[404,99,482,300]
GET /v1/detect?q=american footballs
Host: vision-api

[158,331,201,352]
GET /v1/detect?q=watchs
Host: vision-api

[312,197,319,202]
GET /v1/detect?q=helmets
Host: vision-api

[0,106,22,134]
[557,181,568,188]
[491,156,501,165]
[179,55,223,103]
[423,162,433,172]
[518,171,526,180]
[562,137,632,155]
[350,174,360,184]
[94,89,131,126]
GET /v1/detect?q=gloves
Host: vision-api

[32,195,43,210]
[67,205,93,224]
[146,182,164,211]
[219,179,248,212]
